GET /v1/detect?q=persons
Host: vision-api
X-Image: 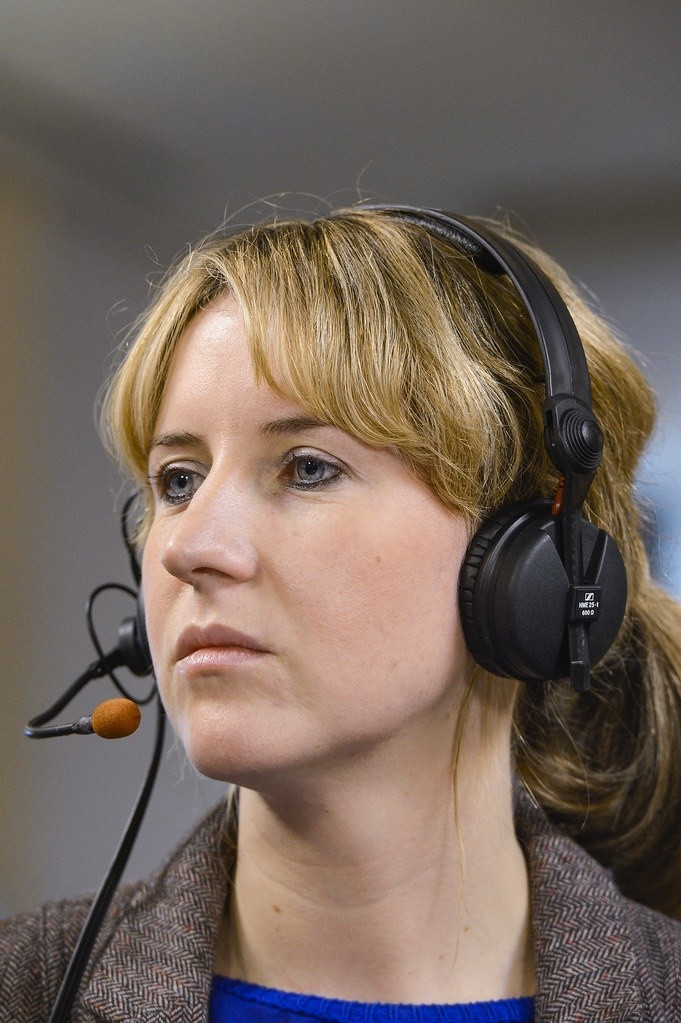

[0,201,681,1023]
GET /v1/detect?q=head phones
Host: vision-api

[136,204,629,686]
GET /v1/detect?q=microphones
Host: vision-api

[24,618,148,740]
